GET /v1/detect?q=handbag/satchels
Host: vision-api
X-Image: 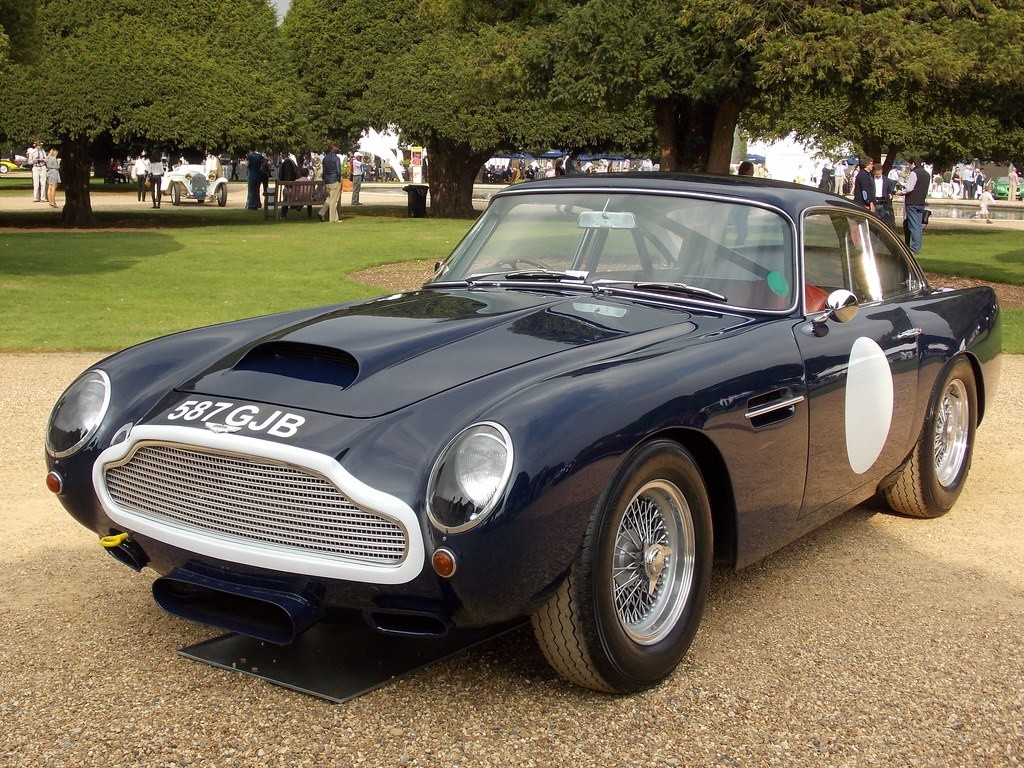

[144,177,151,188]
[922,209,932,224]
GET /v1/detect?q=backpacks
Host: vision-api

[284,158,302,178]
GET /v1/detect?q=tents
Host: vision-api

[490,149,630,161]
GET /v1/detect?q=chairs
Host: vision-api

[748,278,831,315]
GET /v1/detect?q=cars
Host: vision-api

[0,161,18,173]
[220,157,249,182]
[990,176,1024,200]
[153,155,230,207]
[44,173,1016,698]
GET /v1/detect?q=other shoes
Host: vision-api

[336,220,342,222]
[318,213,325,221]
[986,219,992,224]
[50,202,59,208]
[352,203,363,205]
[280,216,286,220]
[150,205,160,209]
[969,211,976,219]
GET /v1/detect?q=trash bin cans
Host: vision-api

[405,185,428,218]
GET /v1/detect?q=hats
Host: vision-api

[354,152,363,157]
[32,139,43,146]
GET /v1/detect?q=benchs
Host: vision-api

[691,243,904,294]
[263,179,341,224]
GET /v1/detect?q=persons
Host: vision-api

[348,151,401,206]
[730,161,755,245]
[483,162,540,184]
[230,151,318,219]
[318,146,342,223]
[1007,167,1019,201]
[25,140,61,209]
[564,147,583,213]
[818,155,984,255]
[104,149,184,209]
[970,185,996,224]
[553,157,565,213]
